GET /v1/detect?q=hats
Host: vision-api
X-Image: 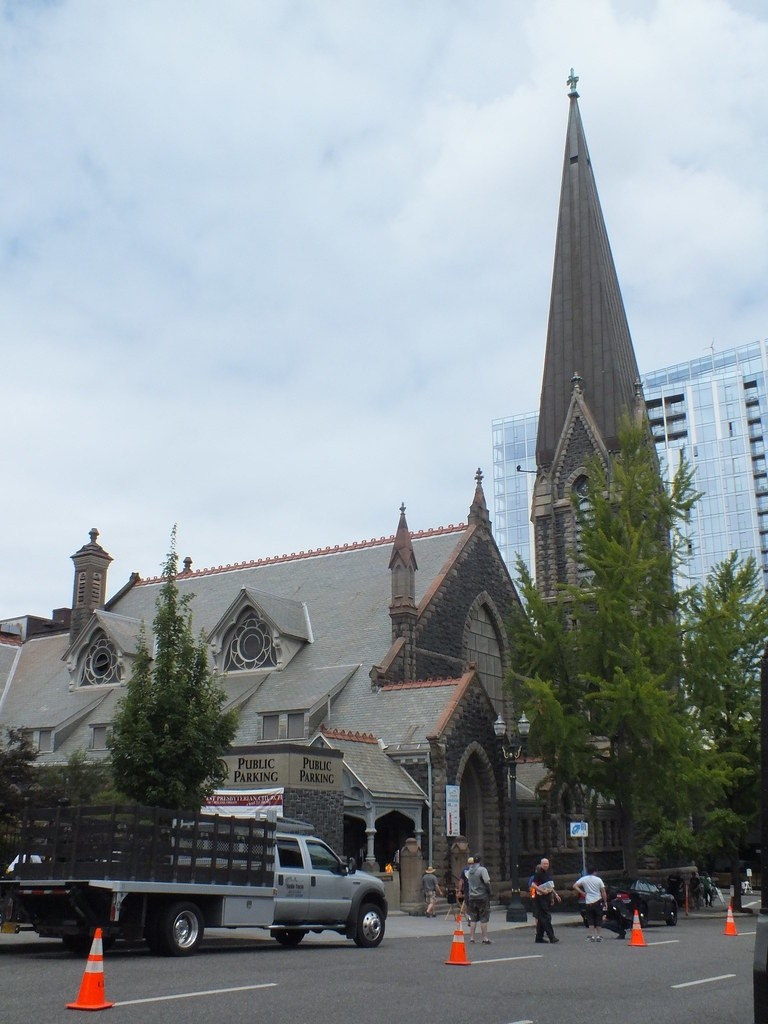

[426,866,436,873]
[468,857,474,863]
[474,855,481,860]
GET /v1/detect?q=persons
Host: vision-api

[459,855,495,944]
[703,872,716,908]
[573,865,608,943]
[602,902,633,939]
[527,858,561,943]
[690,872,701,911]
[420,867,443,918]
[667,873,685,911]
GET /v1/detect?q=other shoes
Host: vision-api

[584,935,595,942]
[432,913,436,917]
[550,936,559,943]
[618,931,626,939]
[482,939,492,944]
[425,911,430,917]
[535,938,549,943]
[596,936,603,942]
[469,940,475,943]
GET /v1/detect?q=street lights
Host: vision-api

[490,710,531,923]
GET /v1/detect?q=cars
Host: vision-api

[576,879,679,927]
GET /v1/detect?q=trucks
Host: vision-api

[5,803,390,957]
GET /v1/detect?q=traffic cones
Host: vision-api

[66,929,115,1011]
[630,909,648,947]
[445,914,471,966]
[723,904,738,937]
